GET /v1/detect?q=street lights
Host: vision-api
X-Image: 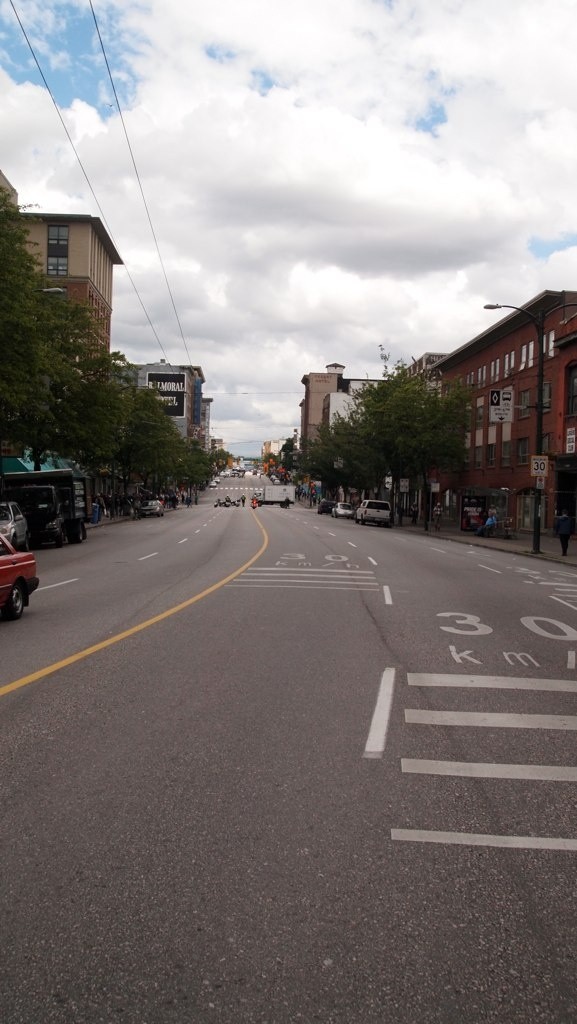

[482,300,577,553]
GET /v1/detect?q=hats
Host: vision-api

[437,502,441,505]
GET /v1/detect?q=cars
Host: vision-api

[318,498,337,514]
[0,532,40,619]
[209,467,247,489]
[356,500,392,527]
[331,502,355,518]
[269,474,280,485]
[139,500,164,518]
[0,501,30,551]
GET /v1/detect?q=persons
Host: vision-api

[225,495,231,502]
[94,488,187,521]
[555,508,575,556]
[295,481,325,509]
[411,501,419,525]
[432,501,444,531]
[186,495,192,509]
[397,498,404,527]
[251,492,258,501]
[285,497,290,509]
[240,493,246,507]
[193,494,198,505]
[474,510,498,536]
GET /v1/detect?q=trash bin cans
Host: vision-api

[89,503,99,523]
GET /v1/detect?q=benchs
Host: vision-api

[487,517,519,540]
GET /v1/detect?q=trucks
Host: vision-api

[0,470,92,550]
[252,485,295,508]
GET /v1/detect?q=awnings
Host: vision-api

[2,458,72,475]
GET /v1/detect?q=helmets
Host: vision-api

[95,493,100,497]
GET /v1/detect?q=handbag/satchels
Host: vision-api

[104,509,107,515]
